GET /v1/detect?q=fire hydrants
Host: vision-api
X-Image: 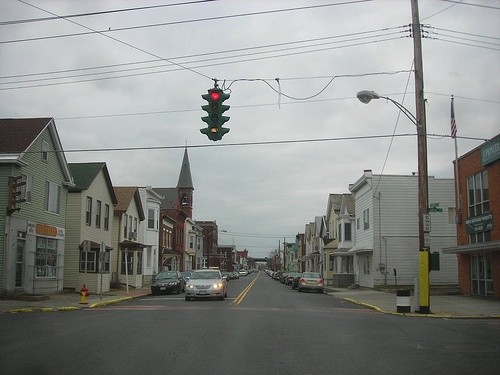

[78,284,89,304]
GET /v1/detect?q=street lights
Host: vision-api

[356,91,430,314]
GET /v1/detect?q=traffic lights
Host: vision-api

[199,88,230,141]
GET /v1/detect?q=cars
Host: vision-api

[181,272,191,282]
[151,270,184,296]
[264,269,302,290]
[299,272,324,293]
[222,269,259,281]
[185,270,227,301]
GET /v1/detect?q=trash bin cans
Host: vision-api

[397,289,411,313]
